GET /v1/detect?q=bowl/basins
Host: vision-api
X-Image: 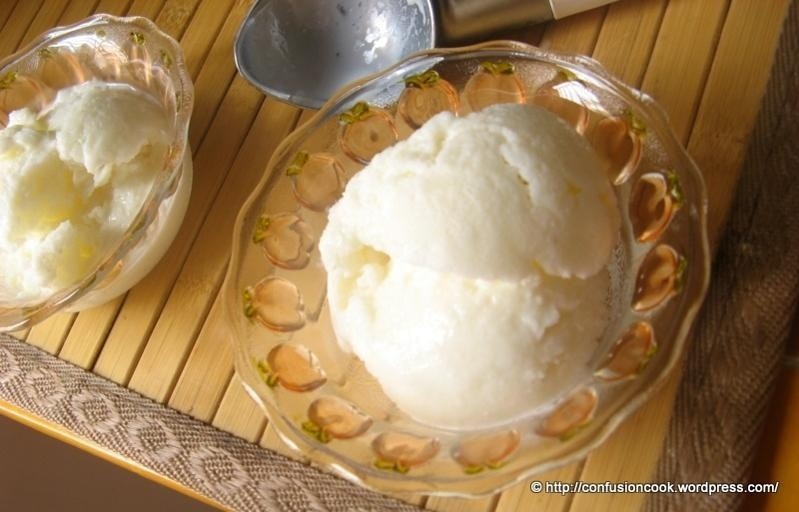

[214,37,715,501]
[232,1,439,115]
[0,12,197,335]
